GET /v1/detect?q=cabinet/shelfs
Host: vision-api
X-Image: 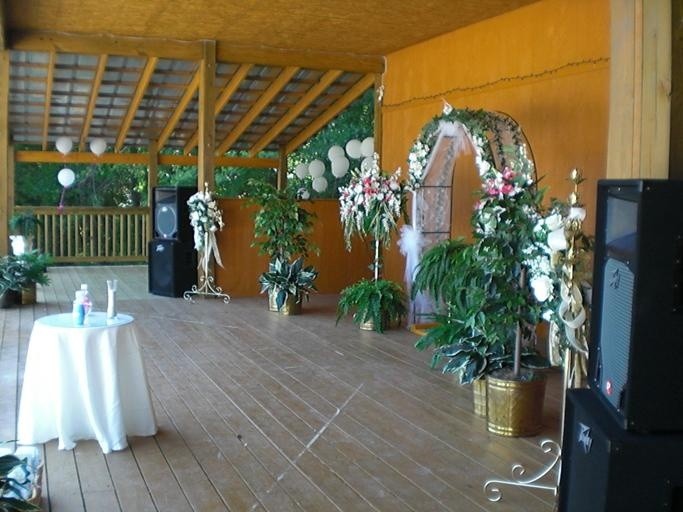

[107,280,118,319]
[72,290,85,326]
[80,284,91,315]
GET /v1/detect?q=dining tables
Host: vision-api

[242,178,317,315]
[0,256,29,308]
[336,279,406,330]
[259,260,316,315]
[438,319,548,436]
[415,239,518,415]
[9,211,40,255]
[13,250,55,304]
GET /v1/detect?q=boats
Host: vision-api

[153,186,198,241]
[558,385,683,512]
[584,179,683,429]
[148,241,198,298]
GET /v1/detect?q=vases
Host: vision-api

[406,142,431,191]
[188,191,222,250]
[336,153,406,242]
[474,169,548,212]
[528,209,586,305]
